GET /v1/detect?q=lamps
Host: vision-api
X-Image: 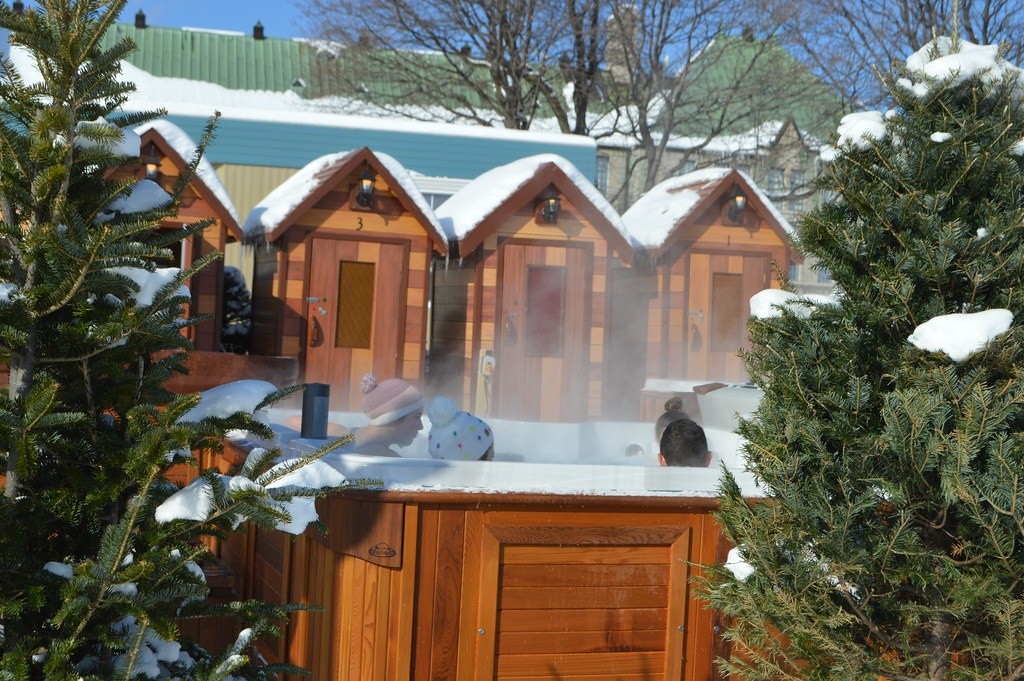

[140,142,163,183]
[727,184,748,224]
[355,169,377,207]
[540,191,561,224]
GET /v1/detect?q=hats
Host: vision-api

[428,396,494,461]
[361,373,425,427]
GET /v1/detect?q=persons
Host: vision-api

[426,395,494,461]
[655,396,693,446]
[657,418,711,467]
[285,372,426,457]
